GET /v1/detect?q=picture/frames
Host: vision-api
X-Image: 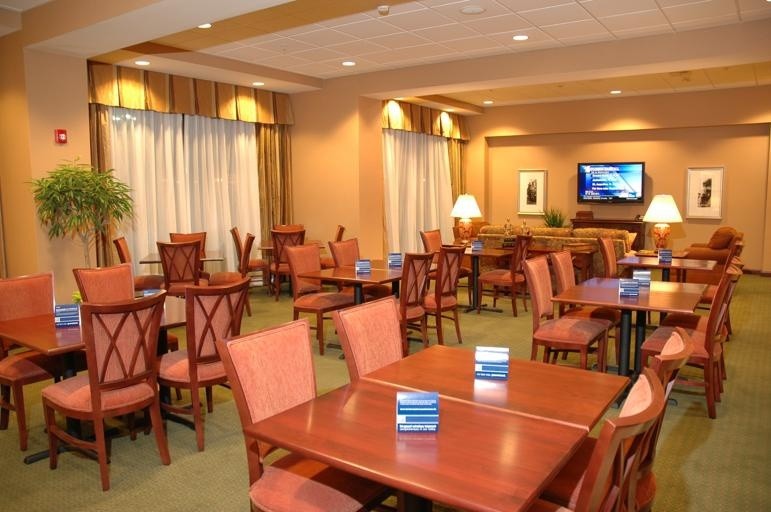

[517,167,549,216]
[686,166,724,220]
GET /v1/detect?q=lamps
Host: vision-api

[450,190,483,244]
[644,194,684,252]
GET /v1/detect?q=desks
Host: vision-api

[362,344,632,433]
[242,378,588,510]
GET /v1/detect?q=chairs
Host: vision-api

[41,288,168,492]
[213,316,399,511]
[520,256,607,379]
[329,237,390,301]
[70,264,185,407]
[284,244,354,356]
[662,256,744,391]
[526,366,667,509]
[476,234,532,316]
[113,234,253,327]
[550,249,619,370]
[643,267,743,418]
[1,270,90,451]
[145,275,251,455]
[394,251,434,354]
[333,295,406,380]
[689,243,742,342]
[417,230,473,316]
[406,244,469,348]
[542,325,694,511]
[230,224,345,304]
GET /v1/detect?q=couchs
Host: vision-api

[671,227,745,283]
[476,228,634,291]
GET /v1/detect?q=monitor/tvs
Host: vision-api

[577,161,645,203]
